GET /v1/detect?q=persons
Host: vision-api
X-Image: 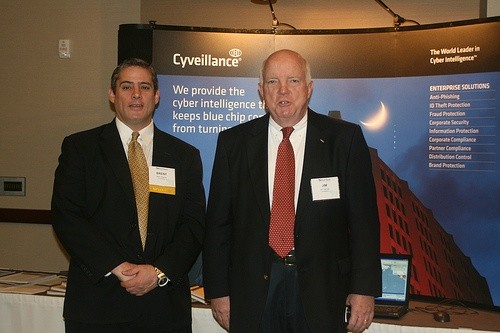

[202,49,382,333]
[51,58,206,333]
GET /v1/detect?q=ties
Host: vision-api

[269,127,301,257]
[128,132,149,249]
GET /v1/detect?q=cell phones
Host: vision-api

[344,305,351,325]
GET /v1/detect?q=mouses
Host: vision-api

[434,311,450,323]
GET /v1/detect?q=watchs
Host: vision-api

[153,266,168,287]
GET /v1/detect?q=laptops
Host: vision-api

[373,253,411,319]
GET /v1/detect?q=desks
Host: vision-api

[0,270,500,333]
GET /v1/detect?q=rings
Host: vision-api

[364,318,369,322]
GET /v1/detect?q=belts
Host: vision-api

[265,246,296,265]
